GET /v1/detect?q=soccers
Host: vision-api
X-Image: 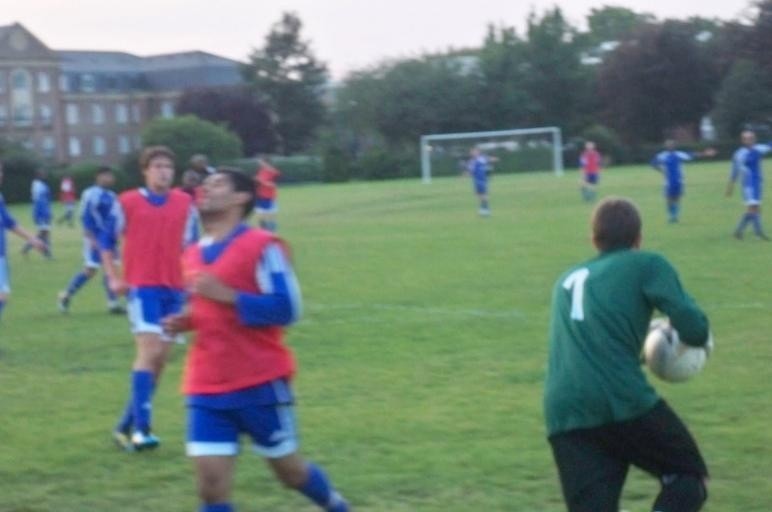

[643,316,714,384]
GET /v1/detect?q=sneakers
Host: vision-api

[56,290,70,316]
[132,432,161,450]
[111,429,133,452]
[106,301,129,317]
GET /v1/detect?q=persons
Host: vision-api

[578,141,600,199]
[544,199,709,512]
[723,125,772,240]
[464,148,499,216]
[650,137,718,222]
